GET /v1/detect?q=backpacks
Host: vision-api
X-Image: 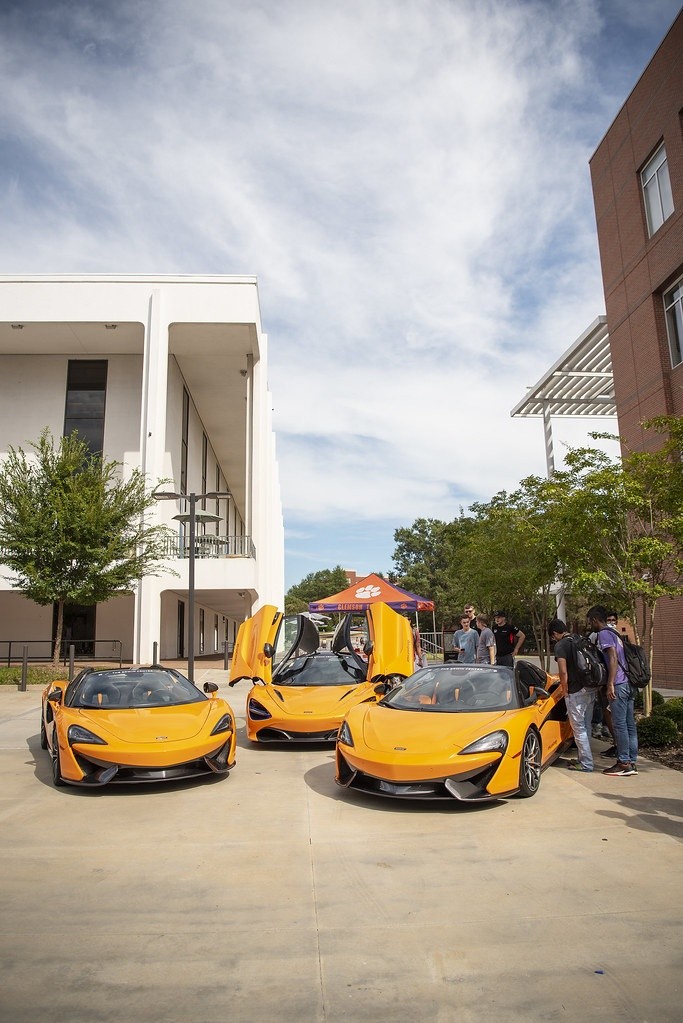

[565,634,608,686]
[602,627,650,688]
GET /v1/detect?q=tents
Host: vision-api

[308,574,439,668]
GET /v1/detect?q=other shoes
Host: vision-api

[570,759,579,765]
[568,763,592,772]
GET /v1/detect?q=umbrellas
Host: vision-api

[172,507,229,558]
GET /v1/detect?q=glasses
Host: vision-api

[464,610,474,613]
[607,619,616,624]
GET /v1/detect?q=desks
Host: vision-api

[193,537,230,558]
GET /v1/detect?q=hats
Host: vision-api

[493,610,506,617]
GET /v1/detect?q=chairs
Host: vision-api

[132,674,168,704]
[84,681,121,706]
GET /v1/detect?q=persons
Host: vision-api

[403,603,638,776]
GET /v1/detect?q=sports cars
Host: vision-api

[41,664,237,787]
[227,603,415,743]
[333,659,578,803]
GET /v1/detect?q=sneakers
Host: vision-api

[600,746,618,759]
[601,759,631,775]
[631,763,638,774]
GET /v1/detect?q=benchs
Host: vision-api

[179,546,210,559]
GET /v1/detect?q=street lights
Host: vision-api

[150,491,232,686]
